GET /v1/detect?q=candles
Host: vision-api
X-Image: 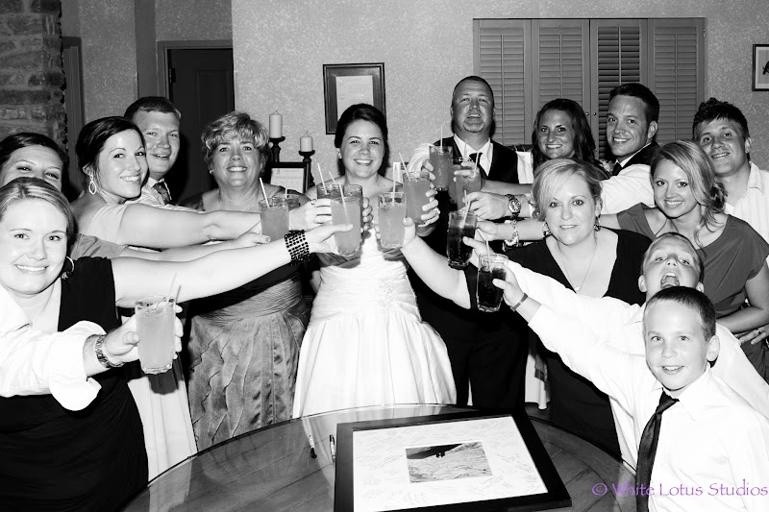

[300,129,313,153]
[269,109,283,138]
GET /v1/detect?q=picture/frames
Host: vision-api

[334,404,574,512]
[321,60,388,136]
[263,161,311,195]
[751,43,769,92]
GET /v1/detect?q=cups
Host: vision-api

[377,192,405,249]
[259,182,362,259]
[429,146,482,226]
[447,211,476,268]
[402,171,431,225]
[476,253,508,311]
[133,296,176,375]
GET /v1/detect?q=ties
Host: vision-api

[611,163,622,179]
[469,152,486,181]
[154,181,170,205]
[633,391,678,511]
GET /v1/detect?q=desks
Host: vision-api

[120,402,638,512]
